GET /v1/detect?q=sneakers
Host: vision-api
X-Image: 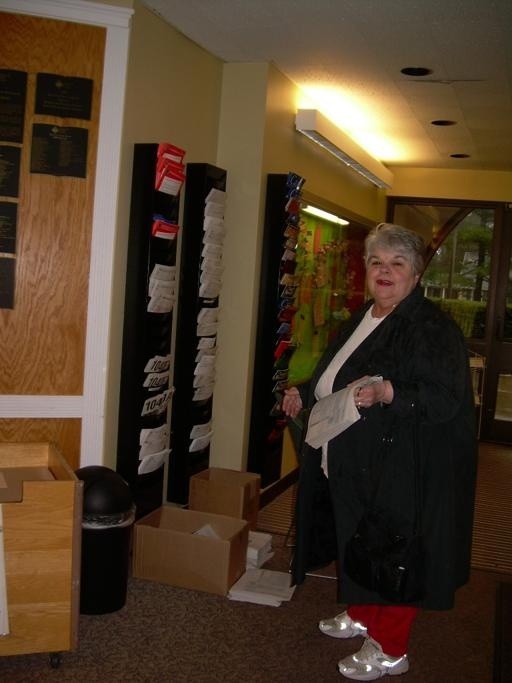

[319,610,369,640]
[336,635,409,682]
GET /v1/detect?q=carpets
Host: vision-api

[257,442,512,574]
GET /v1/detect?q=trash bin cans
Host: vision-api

[73,466,136,615]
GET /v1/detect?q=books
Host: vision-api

[304,373,383,449]
[192,338,217,400]
[200,188,228,299]
[148,264,176,313]
[151,219,179,240]
[189,416,214,452]
[155,140,186,194]
[197,308,219,336]
[138,355,175,474]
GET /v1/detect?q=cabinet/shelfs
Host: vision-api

[246,172,302,488]
[115,143,180,555]
[168,163,227,504]
[1,440,84,669]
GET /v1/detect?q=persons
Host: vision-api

[281,219,478,682]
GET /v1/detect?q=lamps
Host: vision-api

[301,204,350,226]
[295,109,393,188]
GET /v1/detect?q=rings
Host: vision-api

[357,400,361,407]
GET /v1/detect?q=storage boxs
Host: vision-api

[189,467,261,530]
[132,506,249,596]
